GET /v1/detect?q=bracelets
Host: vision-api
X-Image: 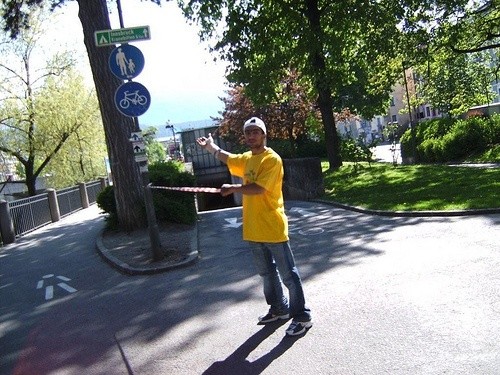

[213,148,222,158]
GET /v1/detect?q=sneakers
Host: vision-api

[260,309,290,323]
[285,320,312,336]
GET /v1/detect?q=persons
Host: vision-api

[196,117,312,336]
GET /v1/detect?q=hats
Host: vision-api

[243,117,266,135]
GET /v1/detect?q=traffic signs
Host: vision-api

[94,25,152,47]
